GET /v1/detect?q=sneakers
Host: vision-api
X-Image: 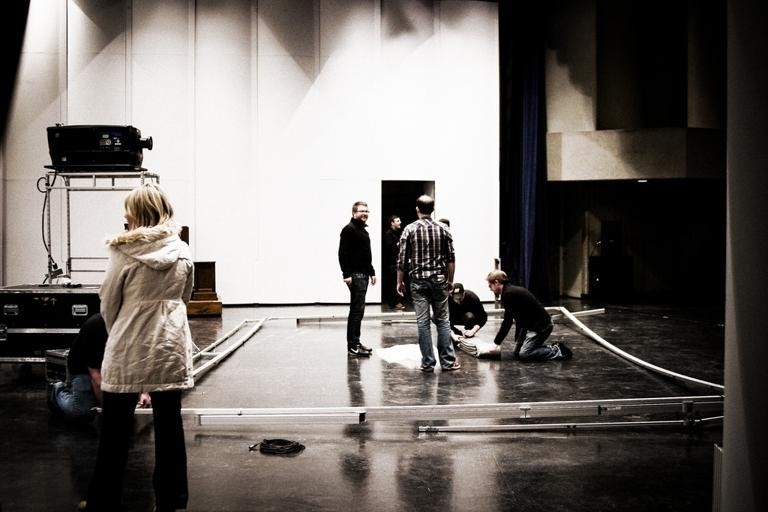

[348,346,370,357]
[357,343,372,351]
[51,382,63,403]
[420,364,434,372]
[392,302,406,310]
[442,362,461,371]
[558,342,572,361]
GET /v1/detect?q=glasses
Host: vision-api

[355,210,369,214]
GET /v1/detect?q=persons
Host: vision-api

[79,187,195,512]
[338,201,376,356]
[47,313,151,430]
[385,194,573,372]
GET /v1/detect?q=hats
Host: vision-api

[452,283,464,295]
